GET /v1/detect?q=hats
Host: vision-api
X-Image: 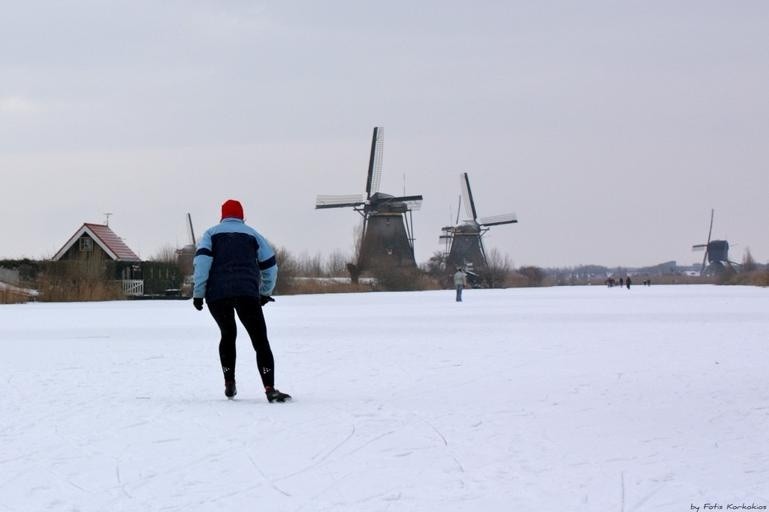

[222,200,244,219]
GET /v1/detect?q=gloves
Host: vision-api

[260,295,274,306]
[193,298,203,310]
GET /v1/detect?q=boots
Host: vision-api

[225,381,237,396]
[266,387,292,403]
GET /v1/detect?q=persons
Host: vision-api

[620,278,623,288]
[644,280,646,286]
[192,200,292,402]
[648,279,650,286]
[454,267,466,301]
[607,278,616,287]
[626,276,631,289]
